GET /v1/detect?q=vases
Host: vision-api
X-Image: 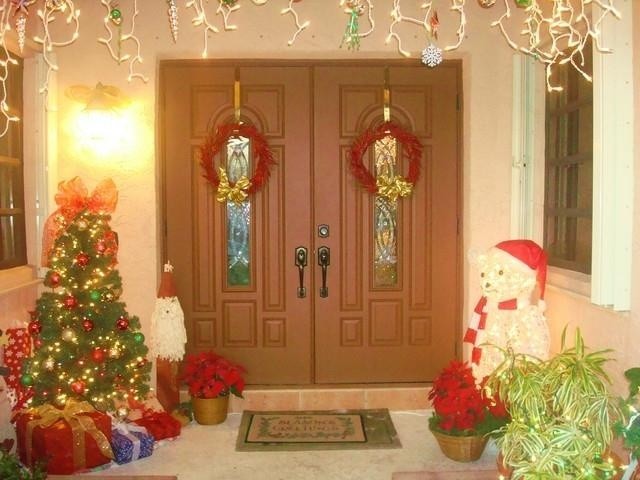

[191,396,229,425]
[429,430,491,461]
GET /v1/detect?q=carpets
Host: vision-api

[235,409,403,453]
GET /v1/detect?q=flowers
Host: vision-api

[176,353,248,399]
[424,360,506,436]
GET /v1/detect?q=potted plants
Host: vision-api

[481,324,626,479]
